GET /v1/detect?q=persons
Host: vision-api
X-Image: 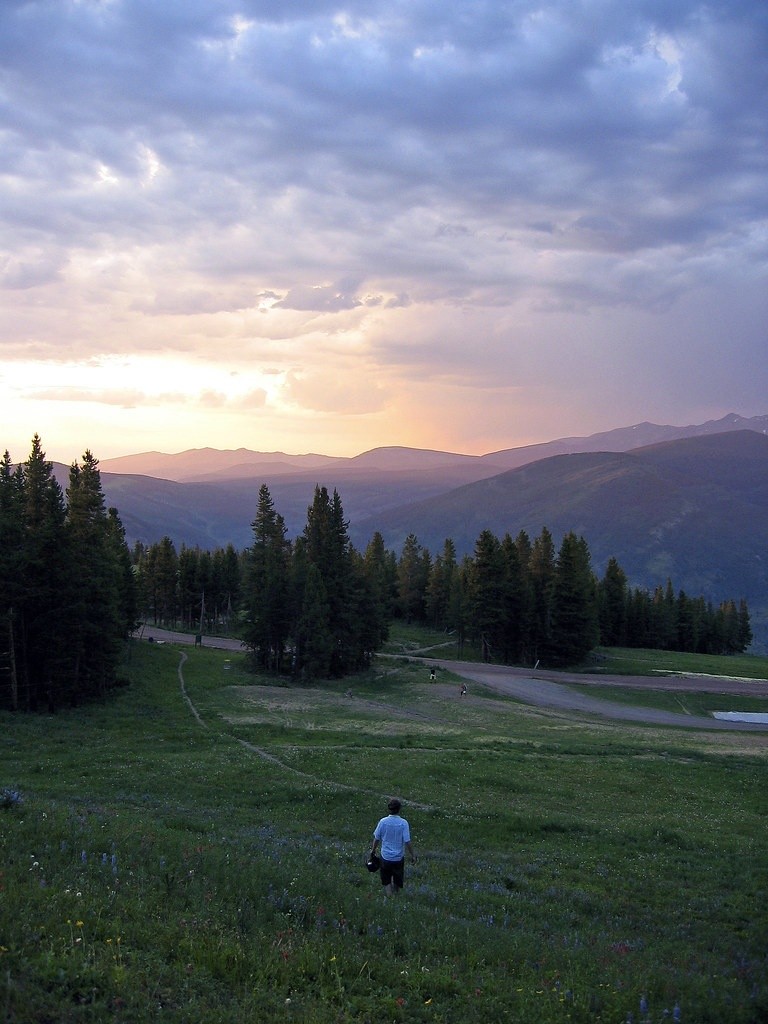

[461,683,467,696]
[371,799,417,899]
[224,653,230,670]
[429,668,436,683]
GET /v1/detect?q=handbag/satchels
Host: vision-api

[365,855,382,873]
